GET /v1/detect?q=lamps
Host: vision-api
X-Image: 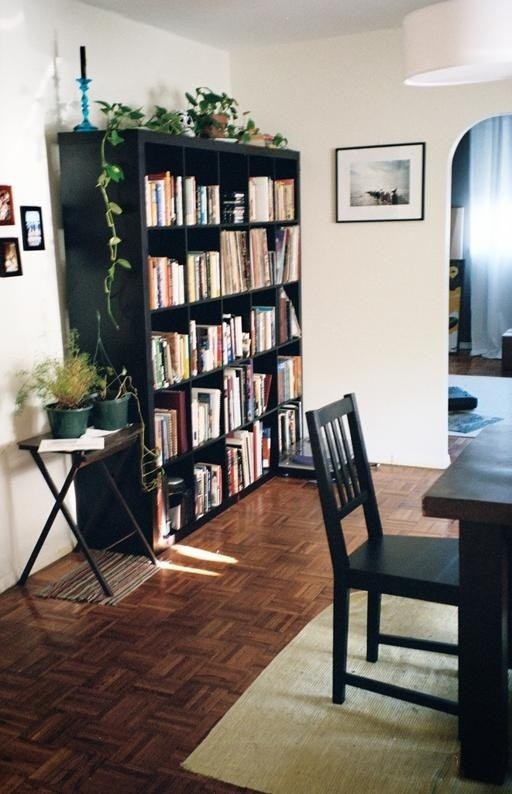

[401,0,512,89]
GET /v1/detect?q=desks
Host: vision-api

[16,421,160,598]
[421,415,512,786]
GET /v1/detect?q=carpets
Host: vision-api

[448,374,512,438]
[32,548,162,608]
[178,589,512,794]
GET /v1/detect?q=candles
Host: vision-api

[80,46,86,78]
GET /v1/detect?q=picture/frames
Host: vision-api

[0,238,22,277]
[0,185,14,225]
[20,206,45,251]
[335,142,426,223]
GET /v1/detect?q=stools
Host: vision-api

[501,328,512,377]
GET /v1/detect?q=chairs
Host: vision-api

[305,391,460,719]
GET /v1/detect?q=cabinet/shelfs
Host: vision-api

[58,127,302,558]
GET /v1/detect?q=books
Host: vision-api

[145,225,300,310]
[155,401,300,534]
[144,170,295,227]
[154,356,301,465]
[150,298,289,390]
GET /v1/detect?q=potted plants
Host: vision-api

[87,309,165,495]
[94,84,289,332]
[14,324,107,438]
